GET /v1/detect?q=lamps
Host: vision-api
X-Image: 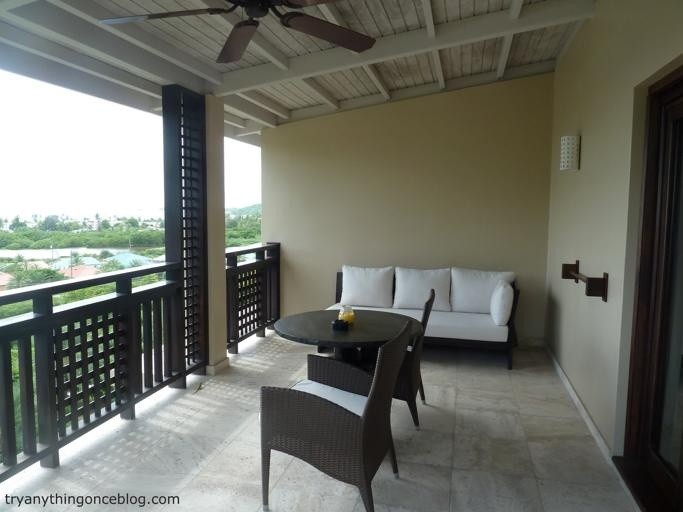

[559,133,581,173]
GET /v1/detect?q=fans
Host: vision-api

[100,1,376,64]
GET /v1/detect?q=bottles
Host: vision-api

[338,306,355,325]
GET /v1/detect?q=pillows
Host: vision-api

[340,265,393,308]
[396,268,452,311]
[490,279,514,326]
[451,266,517,314]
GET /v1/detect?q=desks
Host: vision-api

[274,310,420,366]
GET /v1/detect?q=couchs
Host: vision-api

[328,264,519,370]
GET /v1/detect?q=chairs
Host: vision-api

[261,319,413,512]
[392,288,436,427]
